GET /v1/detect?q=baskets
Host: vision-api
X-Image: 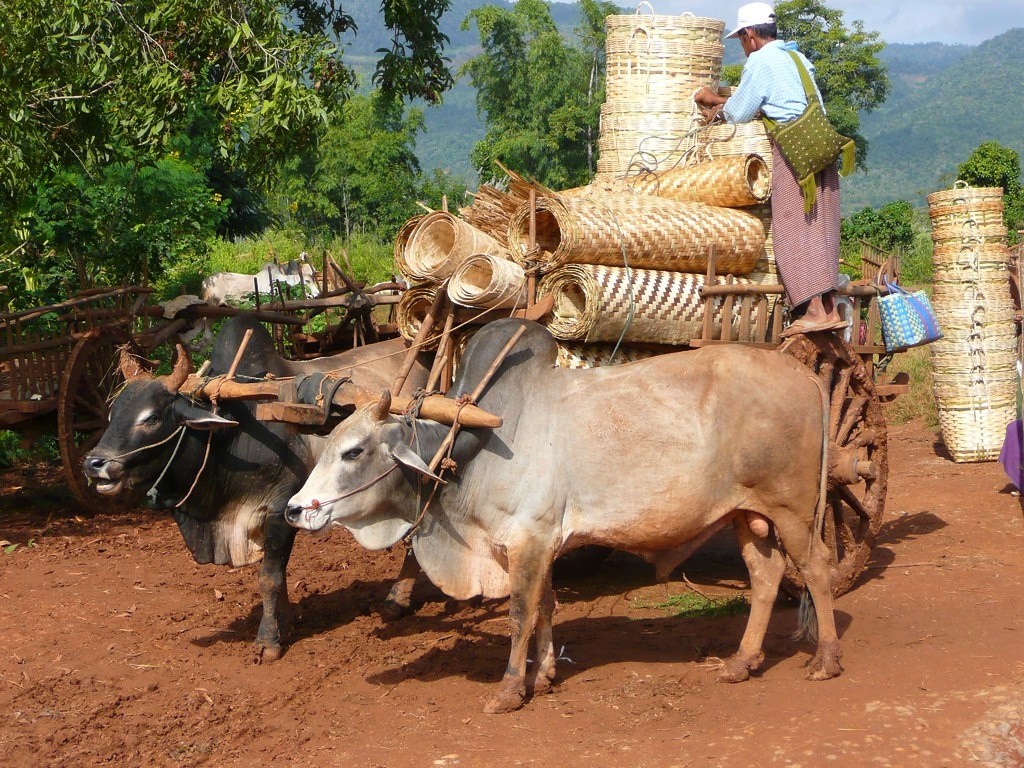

[596,0,774,194]
[925,179,1018,463]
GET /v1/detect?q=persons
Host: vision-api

[693,2,849,338]
[998,359,1024,496]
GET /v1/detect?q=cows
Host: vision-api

[80,313,843,714]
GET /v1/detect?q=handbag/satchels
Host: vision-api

[875,274,944,352]
[760,48,856,215]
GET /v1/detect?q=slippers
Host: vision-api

[778,320,837,339]
[827,320,849,331]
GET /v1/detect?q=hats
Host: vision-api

[724,3,777,39]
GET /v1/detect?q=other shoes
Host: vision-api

[1011,490,1022,497]
